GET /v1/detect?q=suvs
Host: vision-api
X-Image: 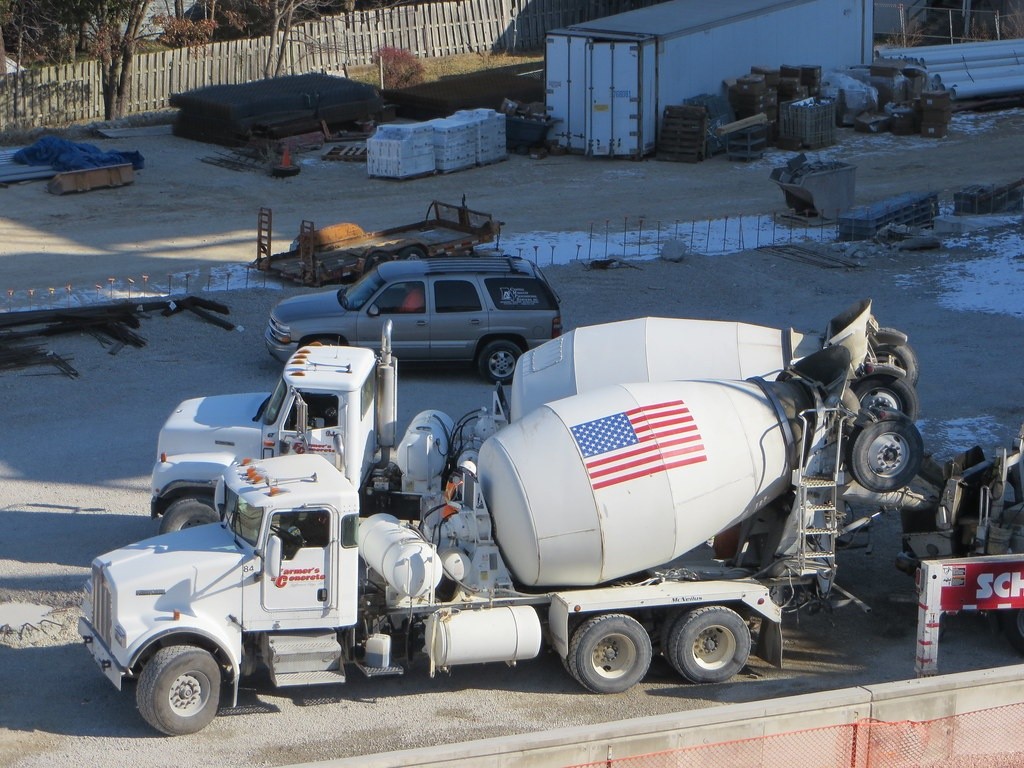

[261,254,562,385]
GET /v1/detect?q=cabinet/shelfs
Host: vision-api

[727,126,766,163]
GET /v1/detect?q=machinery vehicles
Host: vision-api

[77,346,924,735]
[151,298,919,541]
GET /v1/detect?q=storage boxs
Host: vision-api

[923,108,951,123]
[501,98,518,115]
[921,91,950,108]
[921,123,947,137]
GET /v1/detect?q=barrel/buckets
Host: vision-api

[365,633,391,667]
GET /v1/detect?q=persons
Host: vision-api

[290,396,338,428]
[283,511,329,560]
[391,282,423,313]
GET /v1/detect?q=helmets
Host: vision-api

[460,460,477,475]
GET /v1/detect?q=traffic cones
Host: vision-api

[280,144,293,168]
